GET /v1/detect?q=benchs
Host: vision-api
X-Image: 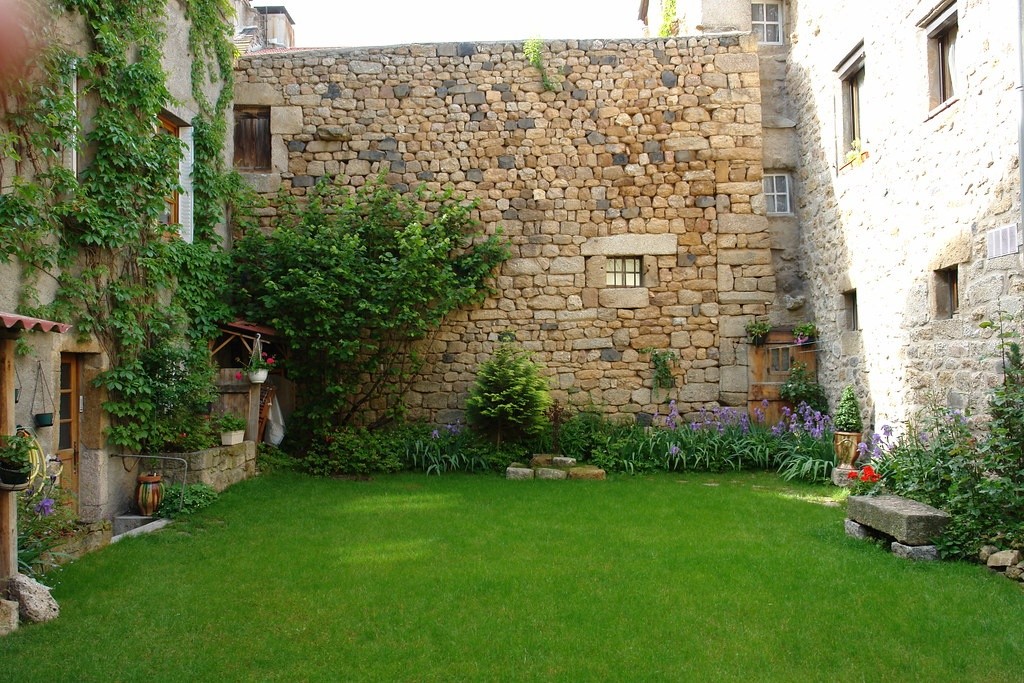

[844,494,952,560]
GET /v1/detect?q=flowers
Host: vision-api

[235,352,276,379]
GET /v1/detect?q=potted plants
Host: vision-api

[215,412,246,445]
[137,438,165,518]
[0,434,38,484]
[831,387,863,469]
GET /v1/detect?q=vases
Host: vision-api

[247,369,268,383]
